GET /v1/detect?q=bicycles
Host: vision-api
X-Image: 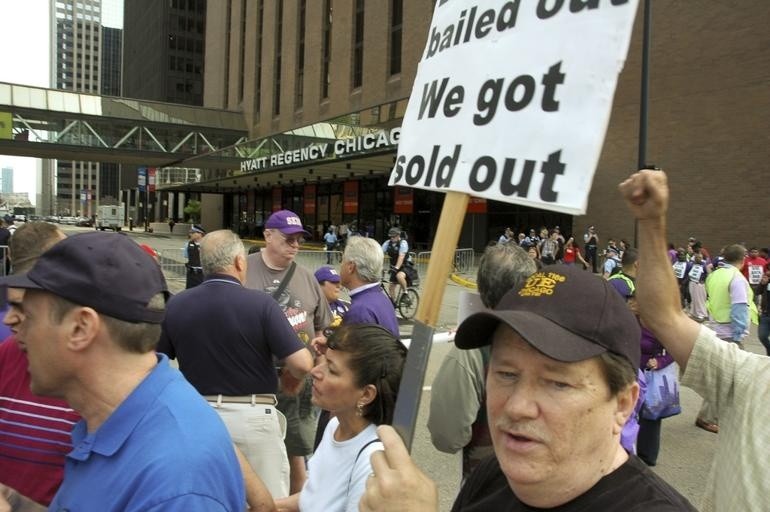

[377,265,419,321]
[333,239,343,262]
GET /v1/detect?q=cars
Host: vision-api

[10,213,93,227]
[234,208,272,226]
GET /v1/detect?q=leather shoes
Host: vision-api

[694,417,719,433]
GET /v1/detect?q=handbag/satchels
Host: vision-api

[640,360,682,422]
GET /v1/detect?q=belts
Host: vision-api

[203,394,276,404]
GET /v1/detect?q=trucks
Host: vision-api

[94,204,124,232]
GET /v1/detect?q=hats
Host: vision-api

[454,264,642,381]
[188,224,206,234]
[315,266,340,282]
[264,210,313,238]
[0,231,170,325]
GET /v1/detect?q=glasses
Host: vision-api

[281,233,307,248]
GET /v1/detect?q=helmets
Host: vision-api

[387,227,402,237]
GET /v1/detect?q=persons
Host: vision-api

[0,209,770,512]
[619,169,769,512]
